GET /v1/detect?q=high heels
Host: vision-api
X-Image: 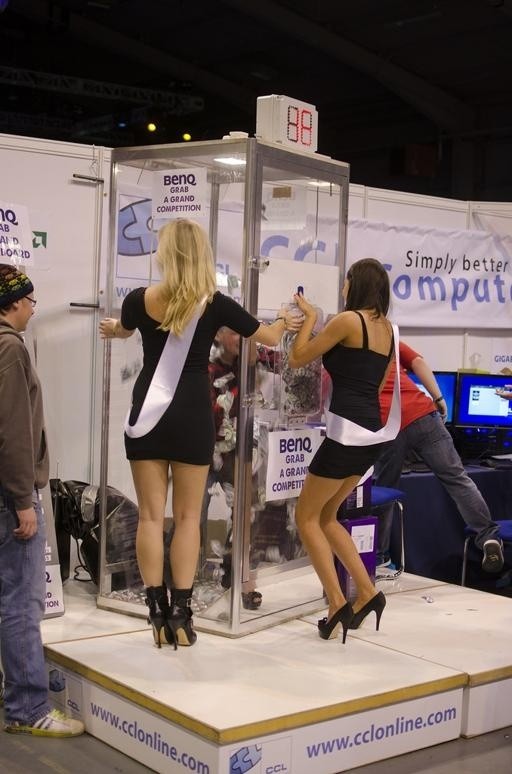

[348,590,387,632]
[317,601,353,645]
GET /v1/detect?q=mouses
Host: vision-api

[479,458,495,467]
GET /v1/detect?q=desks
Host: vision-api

[389,451,510,586]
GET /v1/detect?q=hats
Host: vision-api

[0,263,35,310]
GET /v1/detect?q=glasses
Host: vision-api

[24,296,36,307]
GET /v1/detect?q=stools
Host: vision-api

[359,485,411,589]
[455,513,510,590]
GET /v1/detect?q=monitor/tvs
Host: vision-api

[454,372,512,430]
[409,371,457,427]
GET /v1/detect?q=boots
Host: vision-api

[145,583,179,649]
[165,584,197,650]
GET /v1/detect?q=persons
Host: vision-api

[208,308,325,610]
[495,384,512,401]
[295,259,396,645]
[98,218,304,651]
[0,264,85,737]
[375,343,506,573]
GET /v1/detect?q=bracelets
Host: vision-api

[435,396,444,402]
[275,317,287,328]
[113,322,117,336]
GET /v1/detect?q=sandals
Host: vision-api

[239,590,263,611]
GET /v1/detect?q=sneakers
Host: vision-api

[480,538,505,574]
[2,706,85,738]
[376,552,392,568]
